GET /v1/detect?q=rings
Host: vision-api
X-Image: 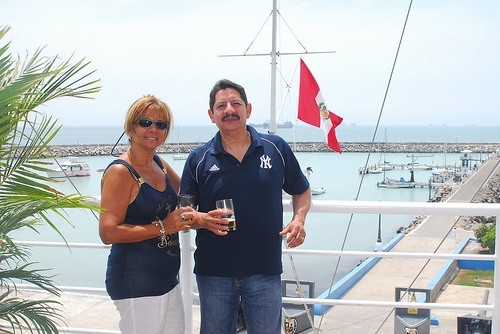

[300,237,304,241]
[181,213,186,221]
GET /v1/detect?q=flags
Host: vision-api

[297,59,343,154]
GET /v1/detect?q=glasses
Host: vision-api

[136,119,169,129]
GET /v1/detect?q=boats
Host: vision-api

[377,169,416,191]
[357,158,431,174]
[45,160,91,180]
[303,165,324,196]
[425,149,488,202]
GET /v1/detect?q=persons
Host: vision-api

[99,96,194,334]
[178,79,311,334]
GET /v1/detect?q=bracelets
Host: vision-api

[156,219,165,238]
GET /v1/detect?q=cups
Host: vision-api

[216,199,237,232]
[178,194,194,227]
